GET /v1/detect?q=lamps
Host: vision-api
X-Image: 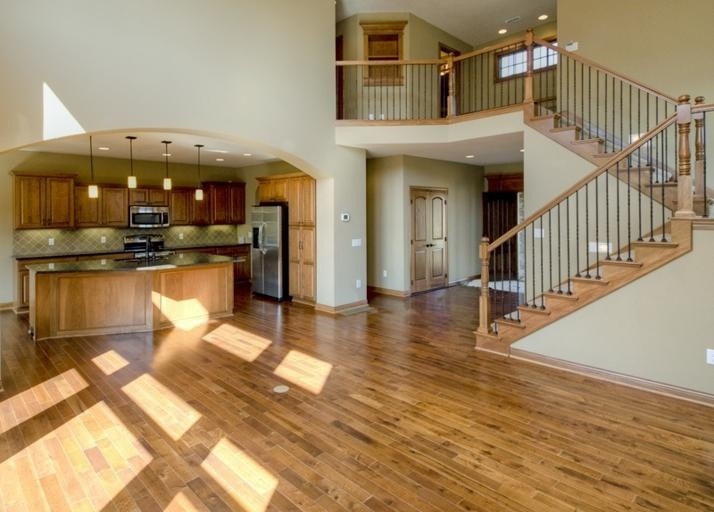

[160,140,173,191]
[193,144,205,201]
[125,136,137,189]
[86,136,99,199]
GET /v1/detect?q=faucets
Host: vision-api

[143,233,154,259]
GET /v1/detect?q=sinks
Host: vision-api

[113,256,165,263]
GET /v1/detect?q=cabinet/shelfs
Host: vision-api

[288,226,315,307]
[201,180,246,224]
[170,186,209,226]
[11,245,252,314]
[254,173,289,203]
[289,171,315,226]
[12,172,74,229]
[358,19,410,86]
[74,185,129,228]
[129,186,168,206]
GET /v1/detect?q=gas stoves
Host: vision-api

[134,246,173,259]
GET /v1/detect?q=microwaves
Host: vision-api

[129,207,171,228]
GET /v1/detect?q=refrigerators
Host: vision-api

[249,204,288,303]
